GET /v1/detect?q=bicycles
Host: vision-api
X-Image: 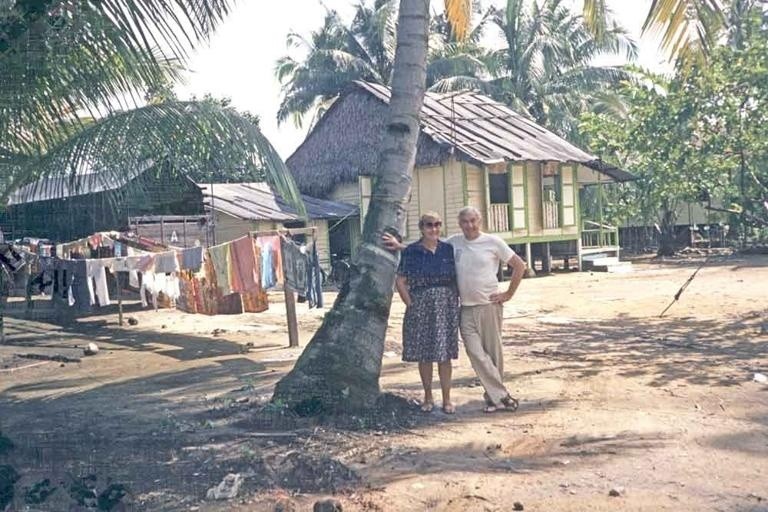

[313,250,352,293]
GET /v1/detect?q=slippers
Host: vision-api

[484,401,496,412]
[501,393,518,411]
[443,404,454,414]
[420,400,434,412]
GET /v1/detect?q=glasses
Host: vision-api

[426,222,439,227]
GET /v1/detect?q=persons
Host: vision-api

[394,210,463,414]
[380,204,528,416]
